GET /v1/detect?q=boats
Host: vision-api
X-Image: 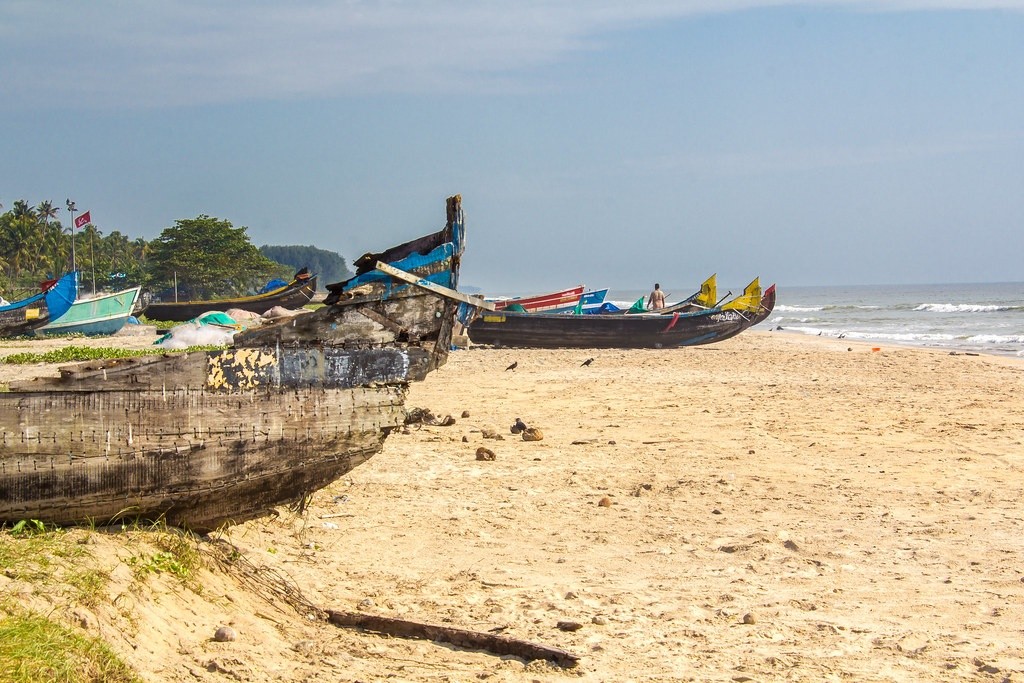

[490,284,586,308]
[136,265,320,321]
[0,193,465,526]
[17,284,143,339]
[465,272,776,350]
[0,268,80,336]
[489,288,612,316]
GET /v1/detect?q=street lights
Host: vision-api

[63,198,78,274]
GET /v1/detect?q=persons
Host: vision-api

[647,283,665,309]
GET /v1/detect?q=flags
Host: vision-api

[74,211,90,228]
